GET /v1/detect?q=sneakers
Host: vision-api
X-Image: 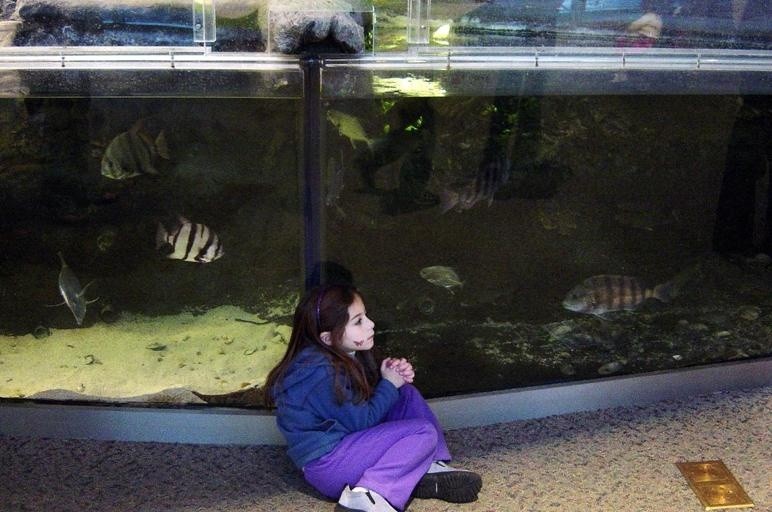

[334,484,395,512]
[411,461,483,503]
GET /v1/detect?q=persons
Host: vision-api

[303,261,380,375]
[264,283,483,512]
[617,0,666,48]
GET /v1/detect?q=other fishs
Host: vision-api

[436,175,499,215]
[153,215,225,265]
[325,168,344,206]
[558,274,680,321]
[100,117,174,182]
[419,266,467,293]
[45,252,100,326]
[326,107,389,153]
